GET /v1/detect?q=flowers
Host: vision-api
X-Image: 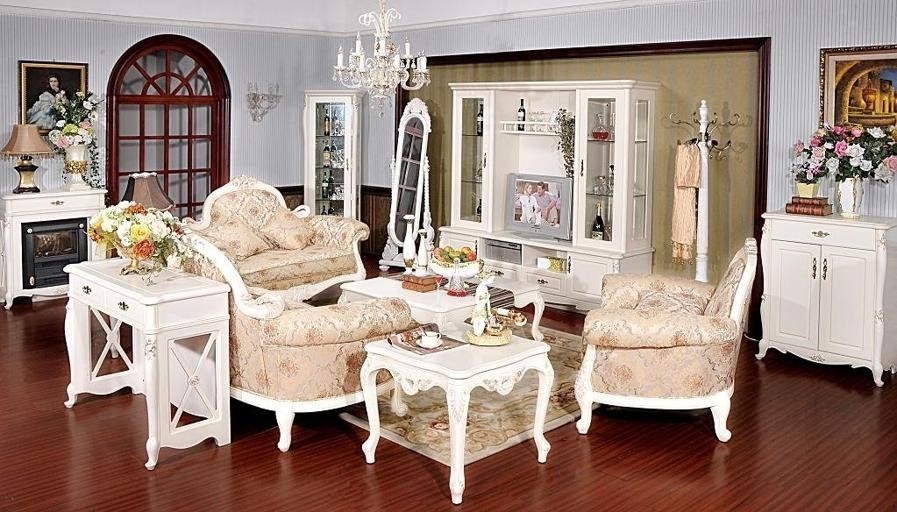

[790,159,821,183]
[88,201,193,269]
[47,90,99,147]
[792,122,897,184]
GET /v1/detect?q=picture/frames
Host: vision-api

[18,59,88,135]
[818,45,897,147]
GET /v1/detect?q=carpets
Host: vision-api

[340,315,599,469]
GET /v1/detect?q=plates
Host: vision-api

[416,338,443,349]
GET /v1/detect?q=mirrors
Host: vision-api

[388,98,432,248]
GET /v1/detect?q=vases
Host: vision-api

[61,144,90,191]
[796,183,819,199]
[838,178,863,217]
[121,253,148,276]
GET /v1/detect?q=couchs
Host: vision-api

[177,234,411,453]
[573,237,758,443]
[176,172,370,307]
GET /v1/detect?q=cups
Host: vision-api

[422,331,441,345]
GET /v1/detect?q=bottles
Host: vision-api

[477,104,483,135]
[592,112,609,140]
[328,109,338,216]
[321,108,330,215]
[447,259,468,297]
[477,199,482,223]
[591,202,604,240]
[518,99,525,131]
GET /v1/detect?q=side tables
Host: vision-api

[359,321,554,505]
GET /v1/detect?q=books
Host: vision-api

[792,197,829,206]
[402,274,436,293]
[786,204,834,216]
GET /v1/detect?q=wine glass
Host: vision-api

[604,223,611,240]
[339,121,345,198]
[332,120,341,200]
[527,112,552,131]
[432,273,443,295]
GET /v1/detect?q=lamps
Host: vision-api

[247,82,283,122]
[332,1,431,117]
[122,172,177,212]
[1,123,54,192]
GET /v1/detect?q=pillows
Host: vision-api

[260,207,316,250]
[219,222,269,260]
[634,290,708,318]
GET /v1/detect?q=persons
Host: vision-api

[26,73,68,130]
[532,182,558,226]
[519,183,542,224]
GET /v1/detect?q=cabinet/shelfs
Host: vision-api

[0,189,109,310]
[302,90,366,222]
[756,207,897,388]
[438,78,661,314]
[63,256,231,470]
